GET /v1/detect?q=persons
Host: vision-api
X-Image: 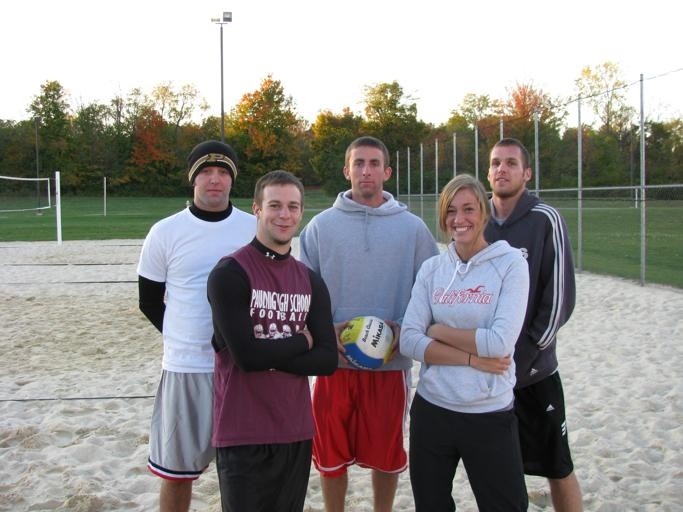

[298,136,444,512]
[398,169,535,511]
[136,139,261,512]
[204,170,342,512]
[480,135,586,511]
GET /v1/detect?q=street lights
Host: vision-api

[211,11,232,140]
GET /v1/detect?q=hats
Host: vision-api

[185,140,239,188]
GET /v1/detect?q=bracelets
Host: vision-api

[468,352,472,365]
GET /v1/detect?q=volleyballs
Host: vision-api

[340,315,395,372]
